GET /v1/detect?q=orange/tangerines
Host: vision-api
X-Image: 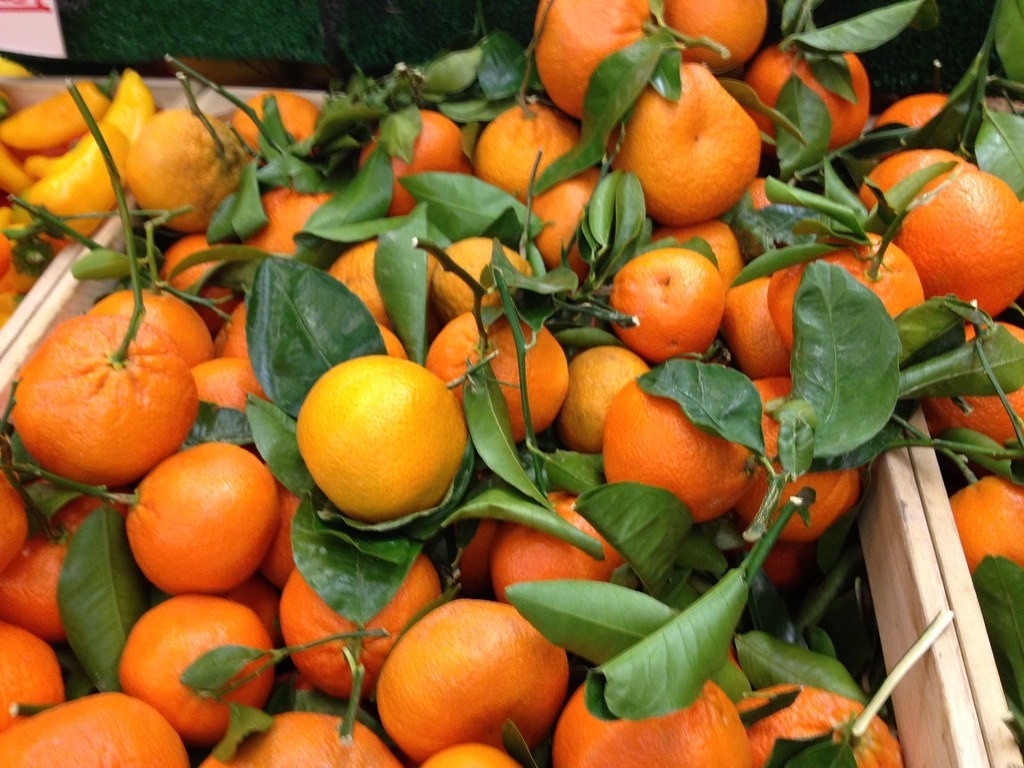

[1,0,1024,768]
